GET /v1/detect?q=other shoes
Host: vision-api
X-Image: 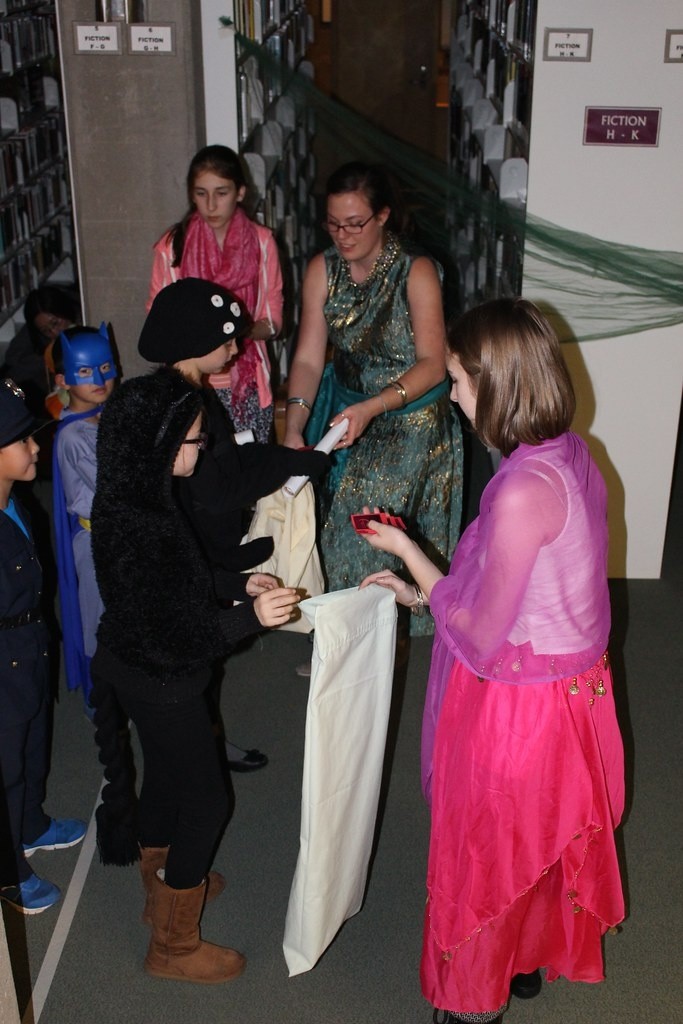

[509,970,542,997]
[432,1007,501,1024]
[297,663,311,676]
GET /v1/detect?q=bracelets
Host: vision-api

[372,395,387,421]
[381,382,406,410]
[287,398,311,411]
[410,582,422,618]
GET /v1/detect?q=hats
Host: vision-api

[0,377,57,449]
[138,278,252,363]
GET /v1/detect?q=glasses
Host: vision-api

[322,214,374,234]
[180,433,210,452]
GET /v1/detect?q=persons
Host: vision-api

[50,324,117,721]
[284,162,465,677]
[0,385,85,914]
[23,289,81,379]
[360,296,625,1024]
[144,145,283,445]
[90,375,299,982]
[141,277,327,775]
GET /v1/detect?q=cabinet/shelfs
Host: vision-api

[1,1,682,578]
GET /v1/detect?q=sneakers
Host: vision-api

[22,819,88,858]
[0,872,61,915]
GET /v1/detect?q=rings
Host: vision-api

[338,413,344,420]
[342,441,346,449]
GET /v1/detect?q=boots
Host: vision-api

[138,846,226,925]
[143,868,247,983]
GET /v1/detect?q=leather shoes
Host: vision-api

[227,749,267,771]
